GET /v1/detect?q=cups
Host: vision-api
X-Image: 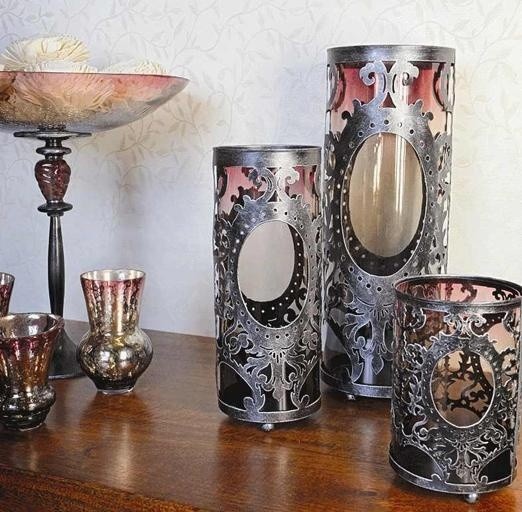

[1,311,66,433]
[76,268,155,396]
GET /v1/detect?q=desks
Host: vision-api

[2,320,521,510]
